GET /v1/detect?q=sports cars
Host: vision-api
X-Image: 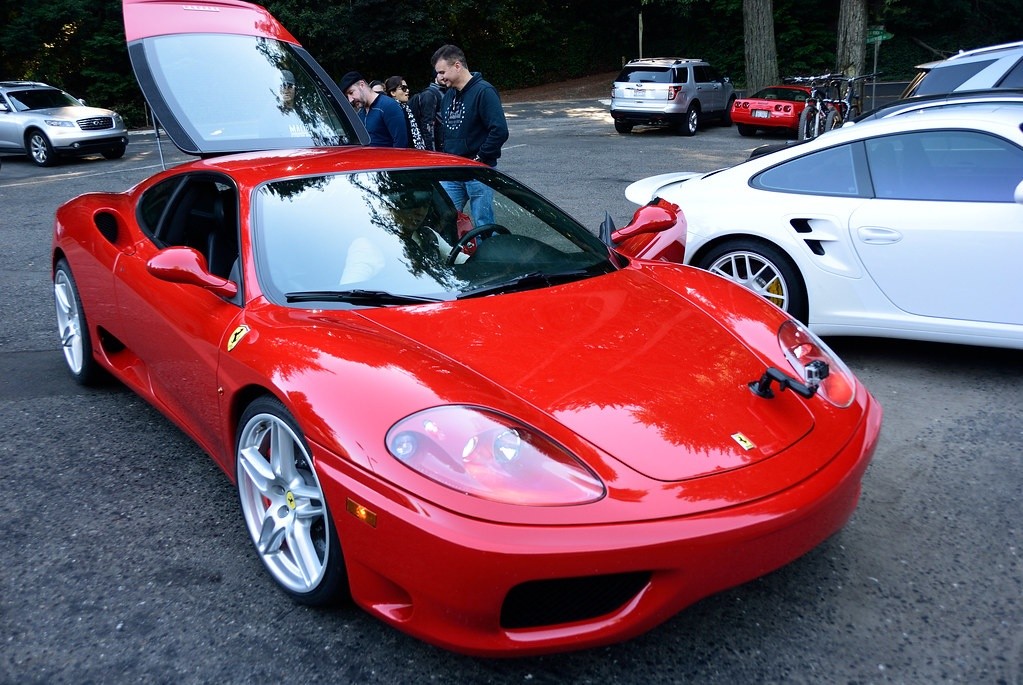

[51,0,882,657]
[730,83,815,139]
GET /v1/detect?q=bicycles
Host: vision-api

[780,58,884,143]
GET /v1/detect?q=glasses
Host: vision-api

[279,83,294,90]
[400,193,433,211]
[395,85,409,92]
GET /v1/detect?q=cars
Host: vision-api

[620,96,1023,349]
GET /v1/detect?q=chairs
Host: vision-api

[208,220,239,275]
[168,181,224,249]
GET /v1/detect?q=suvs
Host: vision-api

[0,79,131,167]
[608,56,740,136]
[897,41,1023,100]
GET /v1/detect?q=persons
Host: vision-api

[341,72,407,148]
[358,80,386,124]
[385,76,439,151]
[433,45,509,244]
[276,70,295,108]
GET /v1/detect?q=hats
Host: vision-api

[339,71,363,93]
[432,69,438,77]
[278,70,295,84]
